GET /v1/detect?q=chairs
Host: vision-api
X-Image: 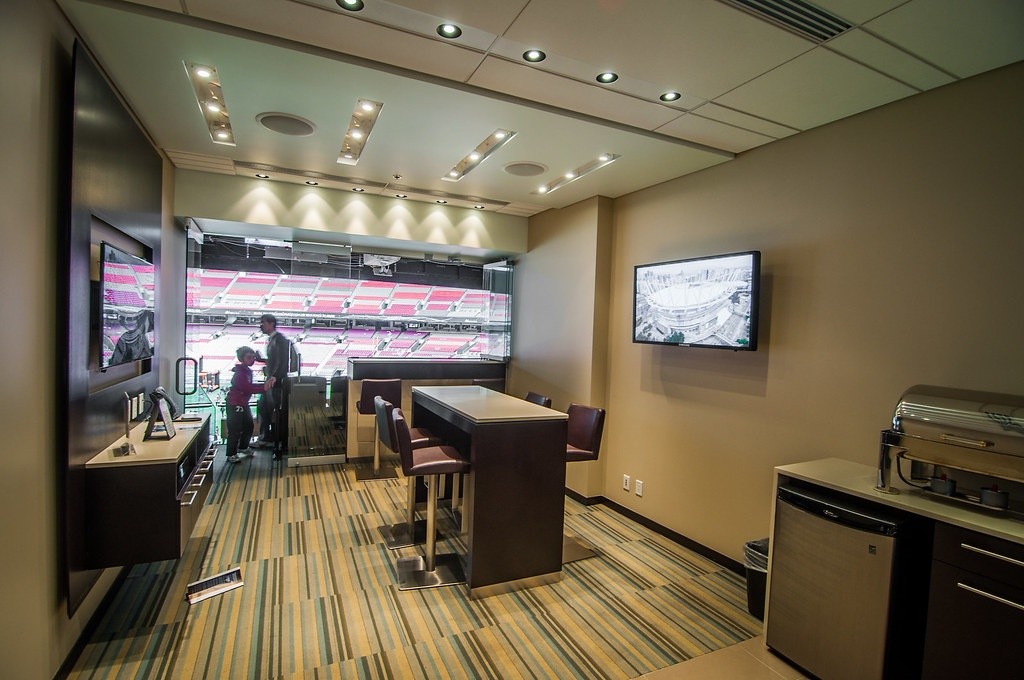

[278,376,348,420]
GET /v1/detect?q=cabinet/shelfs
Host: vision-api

[85,412,219,568]
[921,522,1024,680]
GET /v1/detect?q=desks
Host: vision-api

[410,385,570,600]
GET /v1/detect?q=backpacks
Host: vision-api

[276,335,297,373]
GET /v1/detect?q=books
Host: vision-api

[180,413,202,421]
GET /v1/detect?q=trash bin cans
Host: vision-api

[742,537,772,623]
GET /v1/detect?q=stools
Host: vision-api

[472,378,505,394]
[565,403,606,462]
[525,392,552,408]
[373,396,445,549]
[393,408,470,591]
[355,379,399,481]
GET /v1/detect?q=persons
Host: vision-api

[248,313,292,449]
[225,345,273,464]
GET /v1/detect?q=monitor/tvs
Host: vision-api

[632,251,761,351]
[98,241,155,370]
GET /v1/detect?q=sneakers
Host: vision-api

[227,454,240,463]
[249,440,275,448]
[237,446,254,456]
